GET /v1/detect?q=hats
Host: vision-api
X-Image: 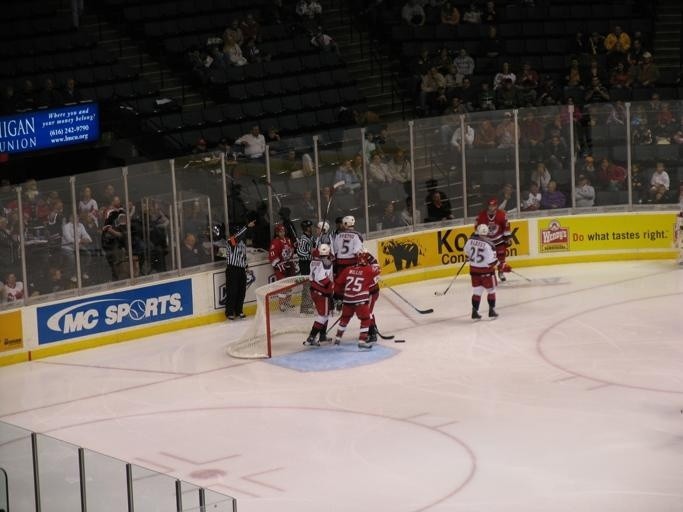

[585,156,596,162]
[643,52,652,58]
[503,111,511,117]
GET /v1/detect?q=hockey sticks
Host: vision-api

[377,332,394,339]
[435,261,466,296]
[318,186,335,237]
[390,288,433,314]
[258,179,299,241]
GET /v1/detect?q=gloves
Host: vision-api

[498,262,513,273]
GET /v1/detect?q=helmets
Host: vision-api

[354,250,370,266]
[275,220,330,234]
[341,215,356,230]
[487,197,498,207]
[318,243,330,258]
[474,223,489,236]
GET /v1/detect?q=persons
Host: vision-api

[224,219,256,320]
[363,253,378,343]
[305,244,332,345]
[463,224,499,319]
[333,252,380,349]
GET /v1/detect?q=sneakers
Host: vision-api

[472,309,482,319]
[227,314,235,321]
[498,272,506,281]
[489,308,498,317]
[239,312,246,319]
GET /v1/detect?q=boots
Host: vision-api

[318,326,333,342]
[306,326,320,346]
[335,324,378,348]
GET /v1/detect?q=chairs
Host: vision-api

[259,0,682,252]
[1,1,258,303]
